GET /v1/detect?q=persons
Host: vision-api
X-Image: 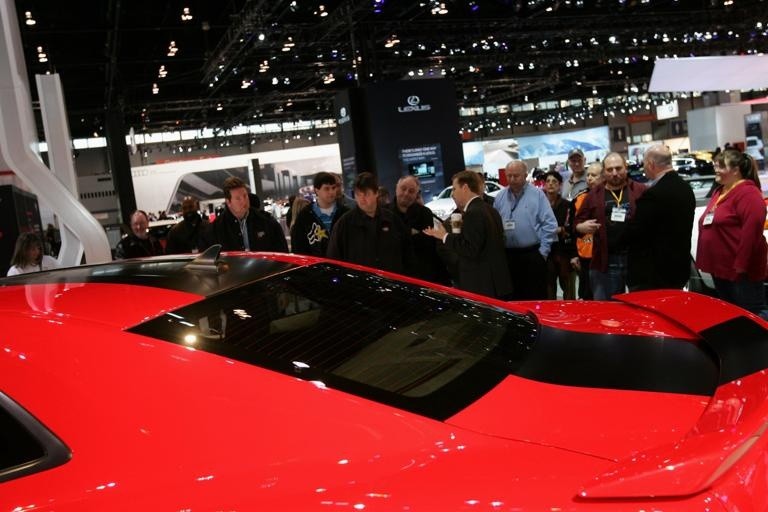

[325,172,413,279]
[381,175,443,285]
[290,172,350,260]
[492,160,558,302]
[329,173,357,209]
[6,232,66,278]
[541,170,577,301]
[696,149,768,314]
[164,196,217,255]
[111,211,164,263]
[422,171,512,302]
[606,142,692,293]
[198,176,289,254]
[561,147,590,201]
[571,163,604,303]
[573,152,644,302]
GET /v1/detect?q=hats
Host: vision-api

[568,148,584,159]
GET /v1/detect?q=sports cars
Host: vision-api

[2,245,768,512]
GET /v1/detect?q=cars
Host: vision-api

[672,158,695,170]
[426,180,505,222]
[684,175,768,292]
[746,136,764,167]
